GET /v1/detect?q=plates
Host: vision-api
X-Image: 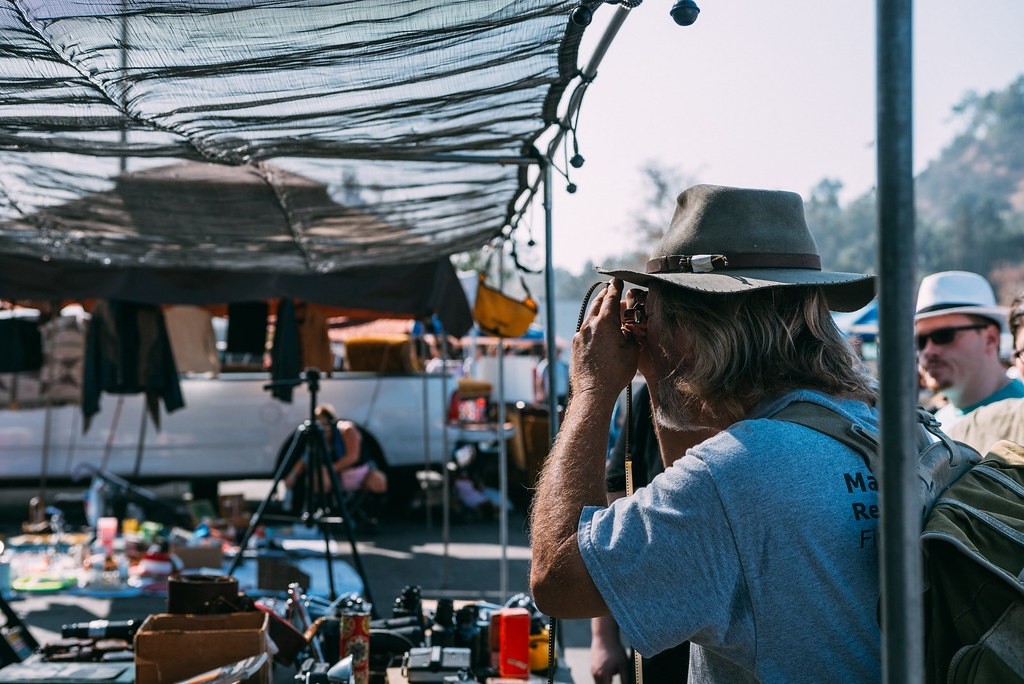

[13,578,76,592]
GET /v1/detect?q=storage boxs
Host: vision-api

[134,612,273,684]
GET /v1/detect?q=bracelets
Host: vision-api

[290,471,298,478]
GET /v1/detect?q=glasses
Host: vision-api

[916,323,987,351]
[1014,349,1024,364]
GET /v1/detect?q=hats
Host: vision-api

[913,271,1008,332]
[594,184,879,313]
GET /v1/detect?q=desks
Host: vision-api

[442,422,519,515]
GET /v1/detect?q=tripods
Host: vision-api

[226,369,386,622]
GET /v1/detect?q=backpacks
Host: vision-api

[771,400,1024,684]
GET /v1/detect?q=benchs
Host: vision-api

[216,467,443,537]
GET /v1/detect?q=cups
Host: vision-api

[168,575,254,614]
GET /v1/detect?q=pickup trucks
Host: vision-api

[1,321,473,539]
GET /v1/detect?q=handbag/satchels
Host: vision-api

[473,234,538,338]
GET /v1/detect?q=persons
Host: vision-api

[287,405,390,527]
[528,183,1024,684]
[413,335,543,488]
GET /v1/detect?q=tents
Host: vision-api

[0,159,474,589]
